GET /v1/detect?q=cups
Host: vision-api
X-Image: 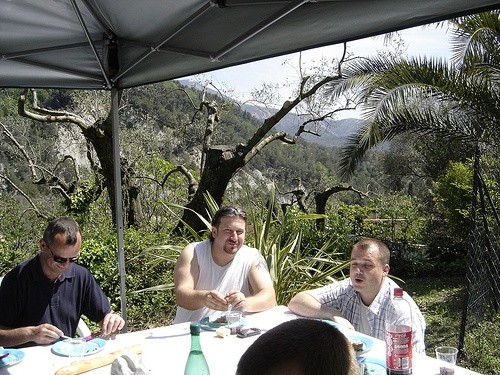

[226,311,242,329]
[435,346,458,374]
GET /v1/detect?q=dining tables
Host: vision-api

[0,305,484,375]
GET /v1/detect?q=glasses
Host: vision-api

[213,207,243,220]
[47,246,79,263]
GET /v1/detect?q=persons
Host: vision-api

[288,239,426,355]
[0,217,126,350]
[235,317,361,375]
[173,205,277,324]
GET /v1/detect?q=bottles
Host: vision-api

[385,287,413,375]
[185,323,210,374]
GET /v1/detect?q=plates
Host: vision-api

[356,356,386,374]
[344,333,374,354]
[53,337,106,356]
[320,319,344,333]
[0,349,25,368]
[199,317,246,331]
